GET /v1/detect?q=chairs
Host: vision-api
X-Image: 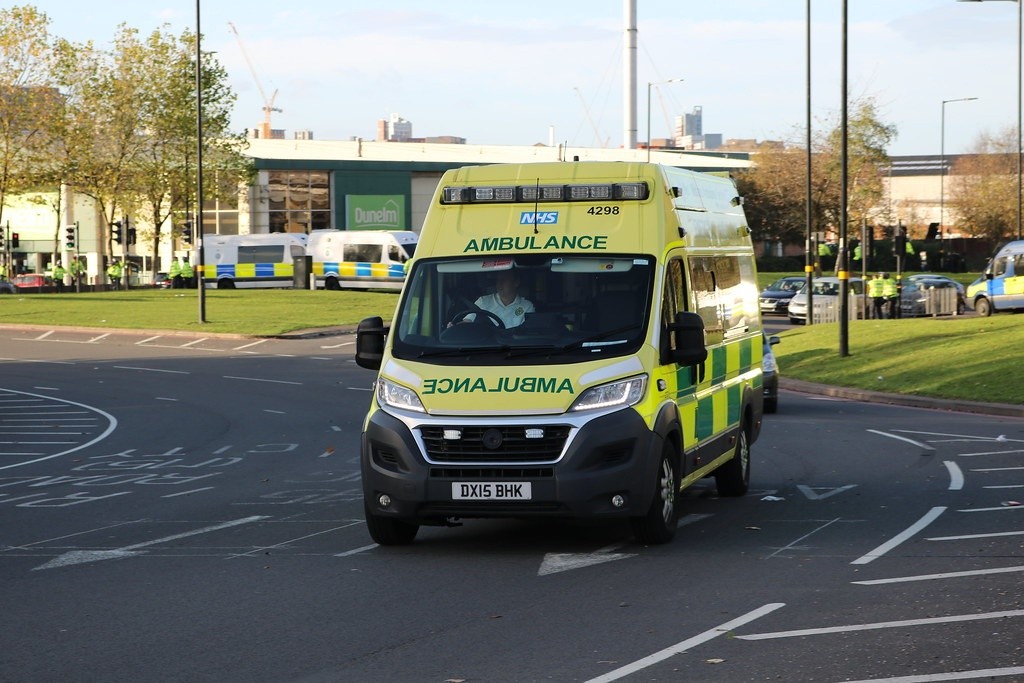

[583,289,641,334]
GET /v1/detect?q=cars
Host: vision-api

[761,325,782,415]
[0,275,18,294]
[12,273,47,288]
[787,277,875,323]
[892,275,966,317]
[152,272,186,289]
[759,276,807,318]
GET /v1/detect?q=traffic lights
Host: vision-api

[66,225,77,251]
[111,221,122,243]
[0,226,5,250]
[13,233,19,247]
[183,222,193,245]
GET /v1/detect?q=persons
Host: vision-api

[866,271,899,320]
[0,260,12,284]
[168,257,184,288]
[106,258,126,291]
[822,284,835,295]
[813,238,945,271]
[446,267,535,330]
[68,254,85,291]
[50,259,67,293]
[181,257,195,288]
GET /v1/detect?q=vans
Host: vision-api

[197,231,311,290]
[305,228,419,291]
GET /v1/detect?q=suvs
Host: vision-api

[964,240,1024,317]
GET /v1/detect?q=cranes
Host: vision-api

[224,19,284,137]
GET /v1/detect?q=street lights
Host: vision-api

[646,79,676,163]
[938,96,980,255]
[952,0,1023,241]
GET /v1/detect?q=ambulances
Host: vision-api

[353,160,764,545]
